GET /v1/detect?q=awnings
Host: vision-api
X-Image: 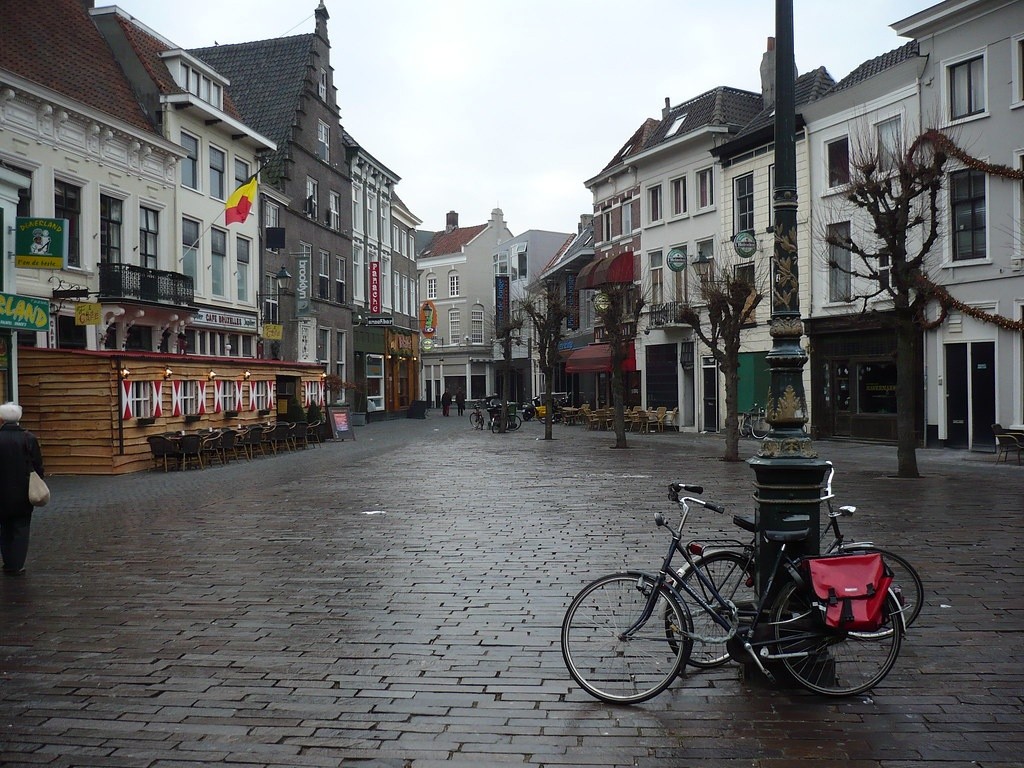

[573,258,607,290]
[565,342,635,373]
[592,251,633,285]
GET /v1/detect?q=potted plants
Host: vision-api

[226,343,232,350]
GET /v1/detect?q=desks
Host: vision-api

[562,407,579,424]
[262,426,271,429]
[171,436,180,439]
[197,433,210,436]
[234,429,246,432]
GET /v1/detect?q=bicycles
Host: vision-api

[739,402,773,440]
[470,399,485,431]
[487,402,522,434]
[658,460,924,669]
[561,481,913,706]
[536,391,573,425]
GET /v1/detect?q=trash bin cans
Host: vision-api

[506,403,520,423]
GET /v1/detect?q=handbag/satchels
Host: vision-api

[29,471,51,507]
[806,553,894,633]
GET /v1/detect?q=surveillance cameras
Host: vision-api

[358,315,362,321]
[466,336,468,339]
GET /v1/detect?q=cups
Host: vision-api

[238,424,242,429]
[209,427,213,432]
[182,431,185,435]
[268,421,271,426]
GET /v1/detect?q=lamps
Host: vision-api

[164,364,173,381]
[244,369,251,381]
[351,314,362,328]
[120,364,131,381]
[256,263,293,309]
[208,368,217,381]
[692,249,730,296]
[321,371,327,381]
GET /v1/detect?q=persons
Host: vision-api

[442,388,452,417]
[455,387,465,416]
[0,403,45,572]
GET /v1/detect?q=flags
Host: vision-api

[225,174,258,226]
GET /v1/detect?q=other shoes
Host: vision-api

[4,565,25,575]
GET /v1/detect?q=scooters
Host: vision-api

[521,396,541,421]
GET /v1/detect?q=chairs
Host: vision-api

[146,420,321,471]
[558,404,678,434]
